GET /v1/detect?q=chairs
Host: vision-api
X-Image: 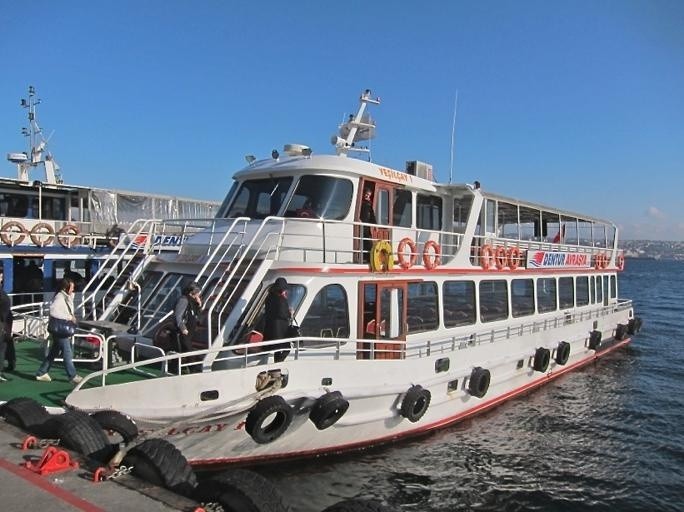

[327,294,590,337]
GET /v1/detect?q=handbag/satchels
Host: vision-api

[290,325,303,343]
[47,318,76,337]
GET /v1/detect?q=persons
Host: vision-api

[174,281,202,375]
[0,283,16,382]
[262,278,291,363]
[36,277,84,384]
[360,187,377,264]
[0,265,3,286]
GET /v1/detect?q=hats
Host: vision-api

[275,278,288,289]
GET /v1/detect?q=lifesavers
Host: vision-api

[59,225,81,249]
[480,245,493,268]
[0,220,27,246]
[509,246,521,268]
[31,222,53,246]
[496,246,507,269]
[398,237,418,269]
[594,254,610,269]
[618,255,624,268]
[423,241,442,271]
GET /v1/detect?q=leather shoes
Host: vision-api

[0,374,12,381]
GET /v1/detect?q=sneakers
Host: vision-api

[69,375,82,383]
[36,372,52,382]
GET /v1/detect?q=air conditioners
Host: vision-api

[406,160,433,181]
